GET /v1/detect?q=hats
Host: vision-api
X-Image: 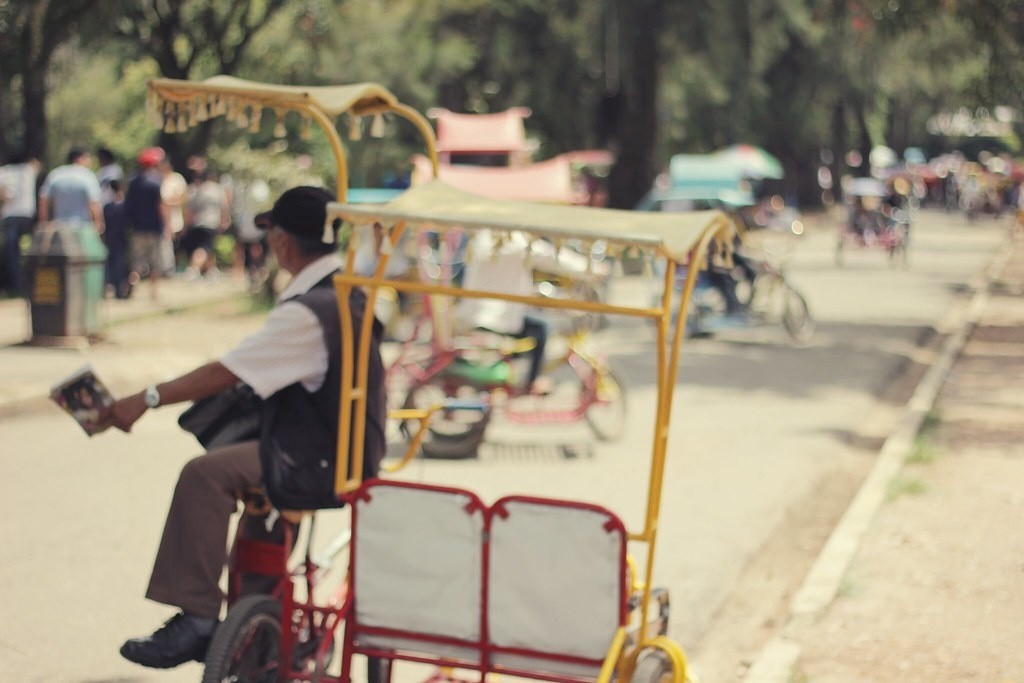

[255,185,341,242]
[137,146,163,168]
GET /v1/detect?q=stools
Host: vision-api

[227,484,318,683]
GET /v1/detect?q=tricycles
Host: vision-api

[201,185,741,680]
[143,69,941,458]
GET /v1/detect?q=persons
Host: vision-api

[707,234,755,313]
[74,384,99,424]
[459,224,611,395]
[112,186,387,670]
[0,146,273,343]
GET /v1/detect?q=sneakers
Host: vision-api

[119,612,211,670]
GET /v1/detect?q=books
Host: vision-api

[50,364,115,436]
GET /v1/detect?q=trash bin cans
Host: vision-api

[23,220,110,338]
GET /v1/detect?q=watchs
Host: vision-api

[144,384,160,409]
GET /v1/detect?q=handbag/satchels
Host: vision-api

[179,384,264,451]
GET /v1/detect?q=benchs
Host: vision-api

[417,279,533,384]
[341,480,669,683]
